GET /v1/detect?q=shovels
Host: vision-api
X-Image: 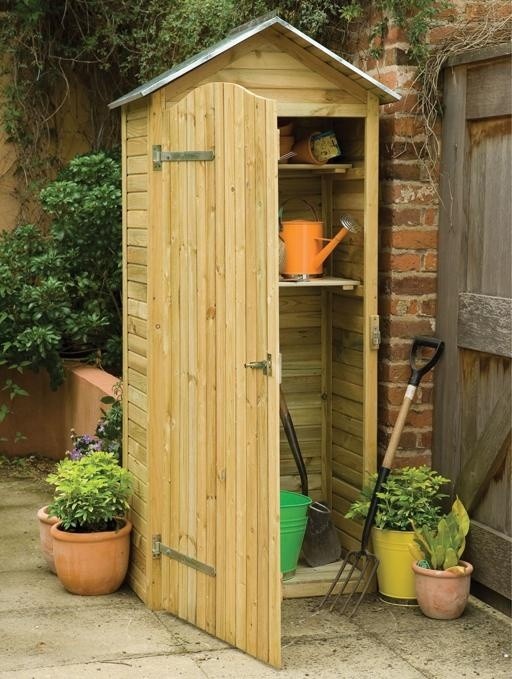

[279,385,342,567]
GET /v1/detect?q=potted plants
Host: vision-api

[46,453,133,596]
[345,463,453,607]
[406,494,475,621]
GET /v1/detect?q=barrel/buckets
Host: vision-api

[280,490,313,578]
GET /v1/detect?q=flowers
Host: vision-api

[63,375,123,462]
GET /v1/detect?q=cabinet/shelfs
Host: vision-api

[278,163,360,291]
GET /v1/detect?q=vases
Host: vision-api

[38,504,63,575]
[278,120,329,164]
